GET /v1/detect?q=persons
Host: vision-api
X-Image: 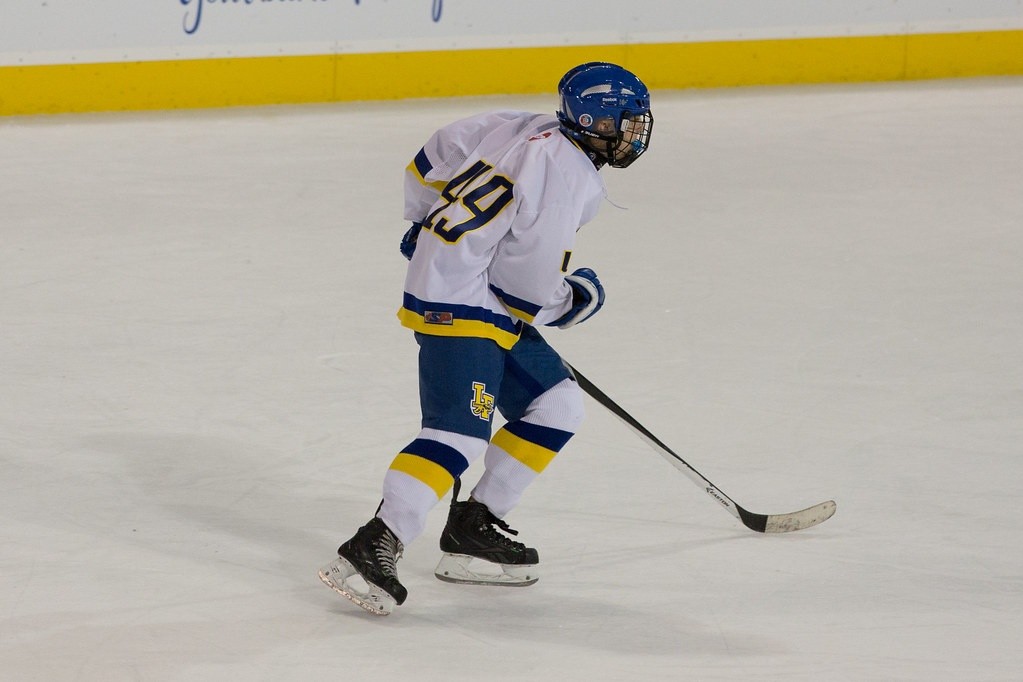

[319,61,654,614]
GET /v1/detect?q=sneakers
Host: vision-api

[318,498,408,616]
[434,477,540,587]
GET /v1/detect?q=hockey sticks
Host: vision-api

[558,356,839,536]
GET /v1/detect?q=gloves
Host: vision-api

[400,221,423,261]
[556,268,606,330]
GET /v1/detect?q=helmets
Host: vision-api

[556,62,654,168]
